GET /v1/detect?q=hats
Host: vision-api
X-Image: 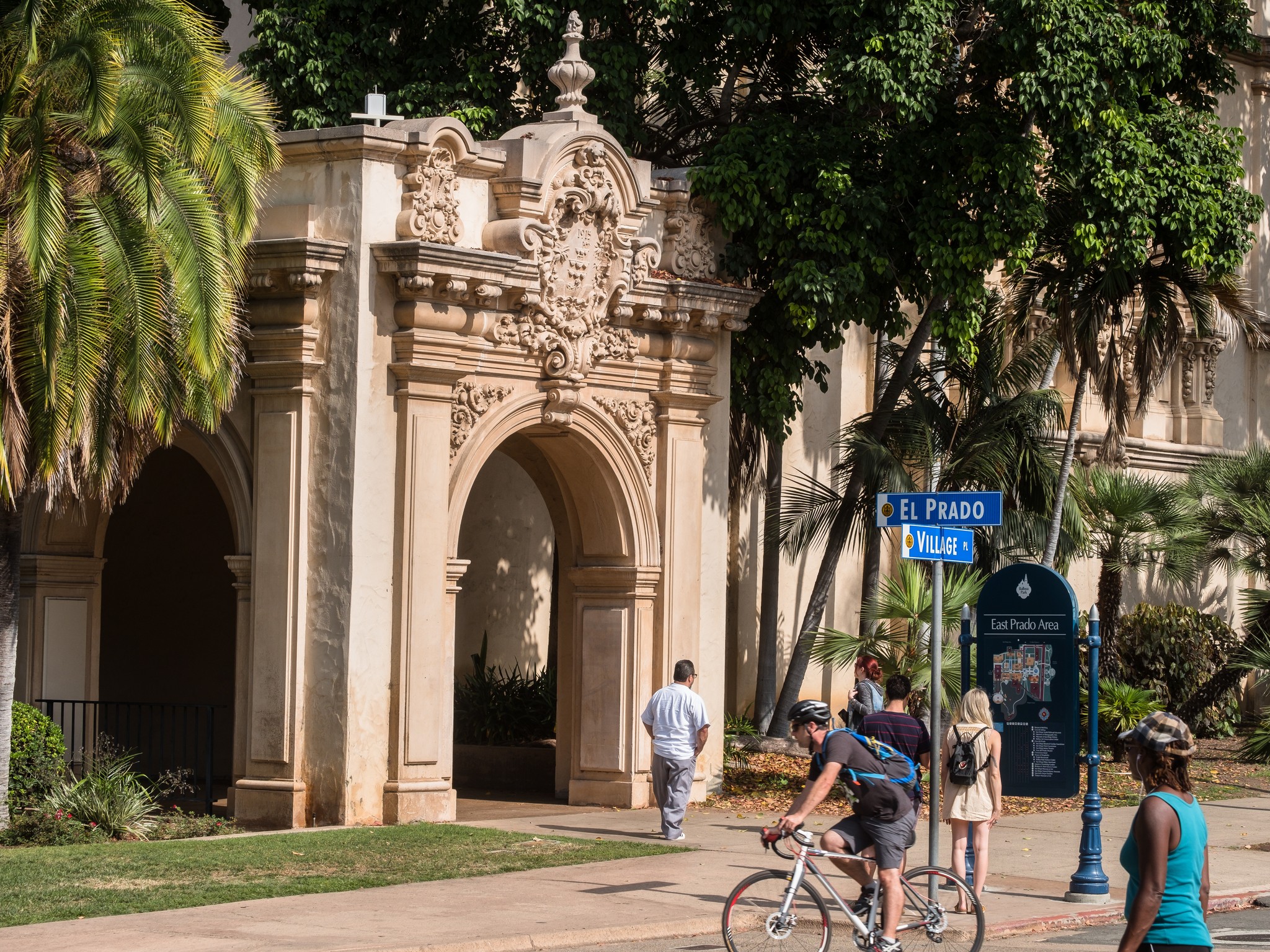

[1117,711,1197,756]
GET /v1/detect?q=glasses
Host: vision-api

[692,674,697,678]
[854,663,862,669]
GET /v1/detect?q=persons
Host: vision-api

[842,655,884,733]
[760,697,920,952]
[856,674,931,916]
[942,688,1001,914]
[641,659,711,841]
[1117,710,1211,952]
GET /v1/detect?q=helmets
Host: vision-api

[788,700,832,724]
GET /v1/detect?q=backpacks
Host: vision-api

[817,727,921,794]
[947,725,991,786]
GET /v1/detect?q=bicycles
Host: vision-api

[718,816,988,952]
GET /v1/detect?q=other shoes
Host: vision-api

[970,903,986,914]
[954,902,968,914]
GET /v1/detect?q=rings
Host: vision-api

[992,821,994,823]
[996,820,998,823]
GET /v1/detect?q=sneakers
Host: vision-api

[851,886,875,915]
[865,936,902,952]
[669,832,686,840]
